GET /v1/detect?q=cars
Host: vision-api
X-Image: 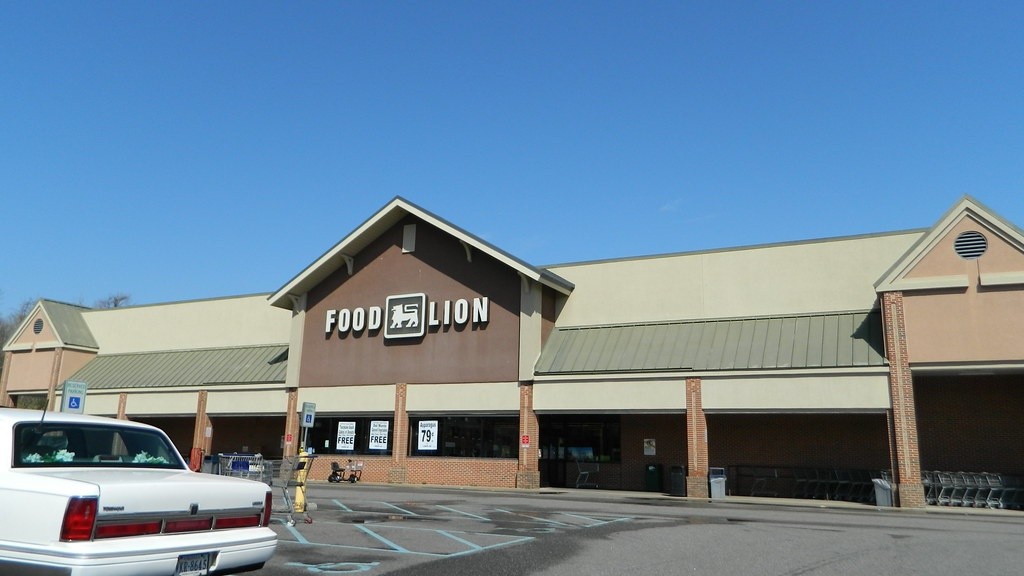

[0,408,279,576]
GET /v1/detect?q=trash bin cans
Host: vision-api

[872,479,891,508]
[202,455,218,474]
[669,466,686,496]
[646,463,662,491]
[708,467,726,498]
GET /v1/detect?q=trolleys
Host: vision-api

[573,457,600,490]
[206,451,318,527]
[789,462,1024,511]
